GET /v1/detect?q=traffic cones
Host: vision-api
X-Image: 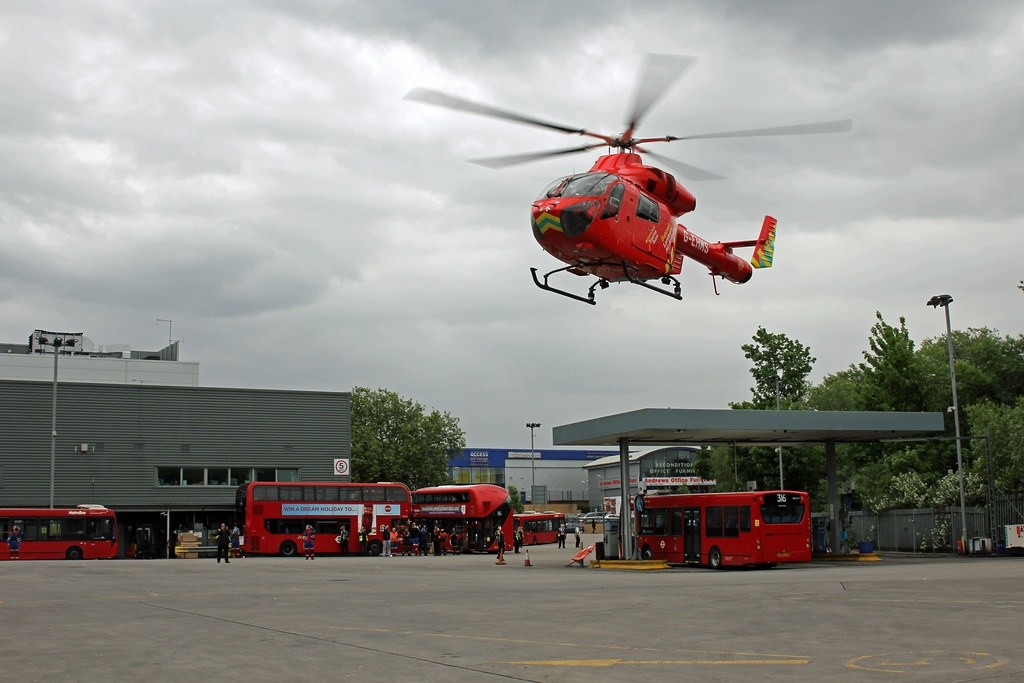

[495,548,507,566]
[523,549,533,567]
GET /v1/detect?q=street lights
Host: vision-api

[925,292,972,557]
[525,421,541,512]
[33,332,79,510]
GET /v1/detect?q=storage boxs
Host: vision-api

[178,533,201,559]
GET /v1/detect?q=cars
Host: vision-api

[565,511,624,534]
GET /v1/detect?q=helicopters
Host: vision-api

[404,52,853,306]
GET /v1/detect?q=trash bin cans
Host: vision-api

[858,541,873,553]
[996,544,1005,554]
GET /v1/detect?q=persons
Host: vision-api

[303,525,316,560]
[401,522,448,556]
[382,524,392,557]
[450,527,460,555]
[339,526,349,556]
[390,527,398,555]
[357,526,371,556]
[575,524,580,547]
[212,523,231,563]
[513,527,524,553]
[230,523,245,558]
[592,519,596,533]
[558,523,566,548]
[7,526,23,560]
[495,526,505,561]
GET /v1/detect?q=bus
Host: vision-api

[350,483,512,552]
[637,489,811,569]
[513,510,566,545]
[0,504,119,560]
[236,481,413,556]
